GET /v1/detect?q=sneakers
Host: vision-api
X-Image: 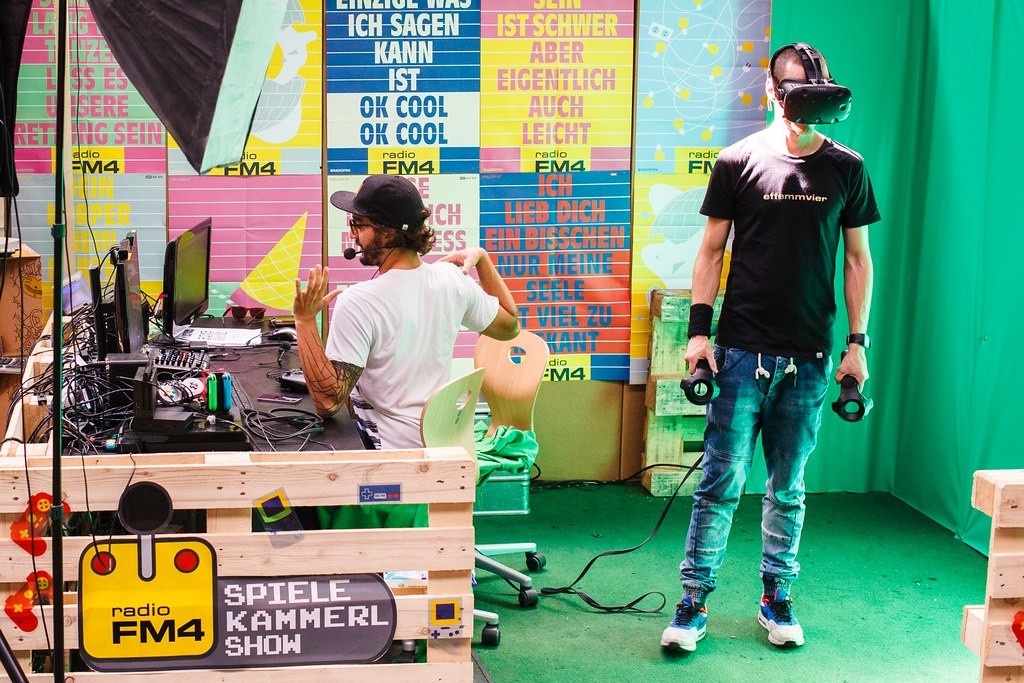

[758,588,804,647]
[661,594,708,653]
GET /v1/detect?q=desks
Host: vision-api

[123,315,366,453]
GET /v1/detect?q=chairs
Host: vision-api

[421,366,539,646]
[473,330,550,572]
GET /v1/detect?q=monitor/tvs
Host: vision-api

[116,230,145,354]
[162,217,213,339]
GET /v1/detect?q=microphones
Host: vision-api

[277,340,292,362]
[344,233,403,261]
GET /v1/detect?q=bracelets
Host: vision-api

[846,334,871,349]
[688,304,714,339]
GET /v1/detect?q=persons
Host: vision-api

[294,174,520,448]
[661,43,881,650]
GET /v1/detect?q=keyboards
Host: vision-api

[229,376,254,412]
[0,357,27,375]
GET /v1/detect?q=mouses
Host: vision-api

[268,327,297,341]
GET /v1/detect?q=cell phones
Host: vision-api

[257,393,303,404]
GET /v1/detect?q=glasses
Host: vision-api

[349,216,383,236]
[221,305,266,326]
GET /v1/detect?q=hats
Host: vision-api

[330,174,425,231]
